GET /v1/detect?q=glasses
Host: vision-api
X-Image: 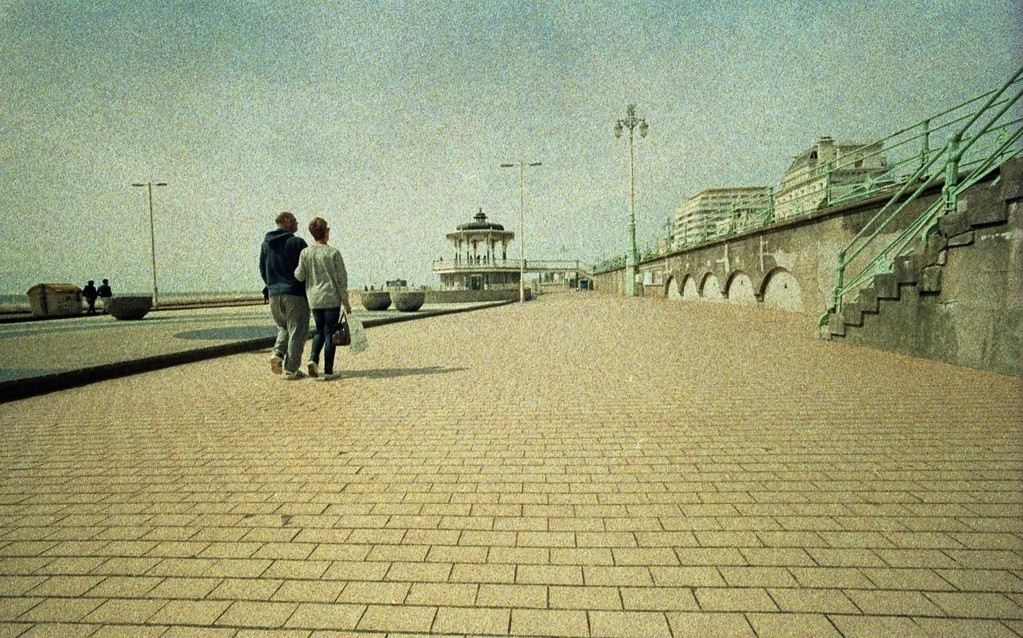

[320,228,330,231]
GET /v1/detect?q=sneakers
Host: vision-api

[282,370,302,380]
[270,354,283,376]
[307,360,319,378]
[324,374,341,381]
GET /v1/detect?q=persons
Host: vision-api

[263,286,269,304]
[364,285,368,291]
[370,285,375,291]
[477,255,486,265]
[258,211,309,378]
[293,217,351,380]
[82,281,97,316]
[96,279,112,315]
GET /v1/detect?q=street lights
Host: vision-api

[135,183,167,308]
[663,223,675,243]
[501,163,542,301]
[613,116,648,296]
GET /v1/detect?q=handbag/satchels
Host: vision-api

[331,311,351,346]
